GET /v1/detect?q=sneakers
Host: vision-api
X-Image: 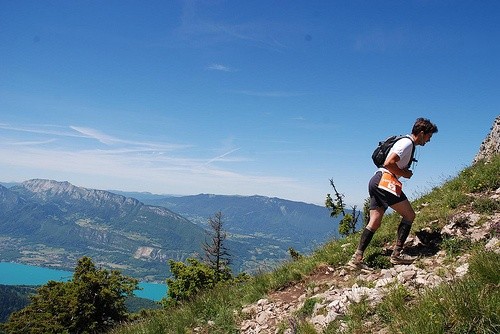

[348,257,373,272]
[390,252,418,265]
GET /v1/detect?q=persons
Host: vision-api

[346,116,439,274]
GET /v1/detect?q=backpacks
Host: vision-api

[371,134,418,170]
